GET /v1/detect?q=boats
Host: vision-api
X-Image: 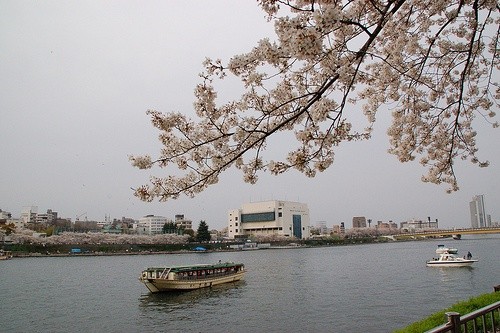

[426,247,479,267]
[138,259,249,293]
